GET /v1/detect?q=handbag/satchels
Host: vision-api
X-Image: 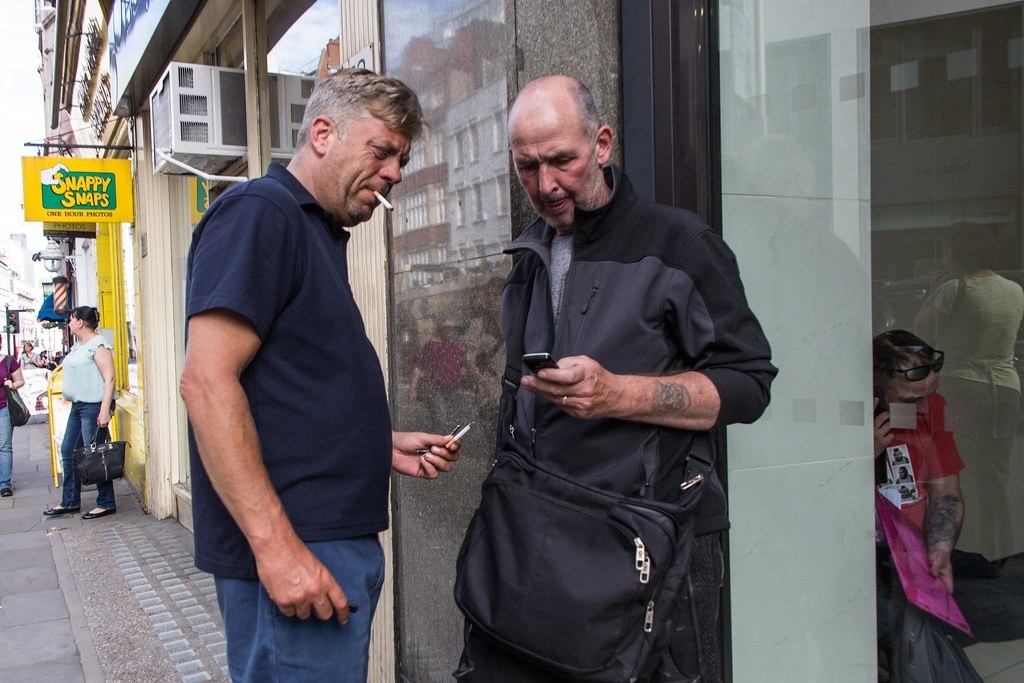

[453,437,708,674]
[2,358,31,427]
[74,424,131,486]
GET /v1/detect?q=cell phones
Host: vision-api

[873,387,889,429]
[523,353,559,374]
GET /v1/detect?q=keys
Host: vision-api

[416,424,460,453]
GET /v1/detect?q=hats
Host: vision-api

[37,347,47,354]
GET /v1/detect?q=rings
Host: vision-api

[562,396,568,406]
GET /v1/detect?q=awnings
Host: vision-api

[37,282,72,328]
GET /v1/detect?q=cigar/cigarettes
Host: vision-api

[445,421,476,449]
[373,190,394,212]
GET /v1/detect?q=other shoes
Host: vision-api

[43,507,80,515]
[82,507,116,519]
[1,488,13,497]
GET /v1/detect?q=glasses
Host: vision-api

[879,348,946,382]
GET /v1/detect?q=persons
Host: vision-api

[180,66,462,683]
[911,220,1024,573]
[887,447,917,503]
[0,333,25,496]
[872,329,1023,682]
[19,343,71,371]
[43,306,119,519]
[407,314,462,434]
[451,75,778,682]
